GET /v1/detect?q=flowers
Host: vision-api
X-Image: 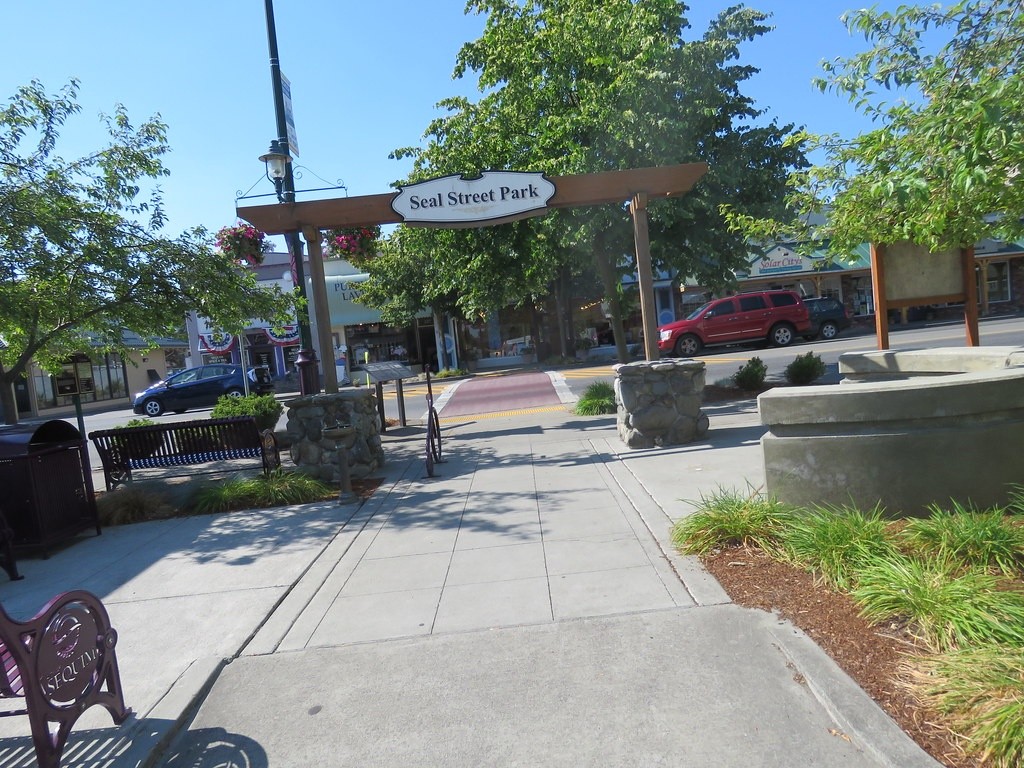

[214,221,275,271]
[322,225,381,262]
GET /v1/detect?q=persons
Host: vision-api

[507,343,518,356]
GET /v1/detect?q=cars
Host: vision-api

[132,363,276,417]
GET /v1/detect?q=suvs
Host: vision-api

[654,288,809,358]
[801,295,850,342]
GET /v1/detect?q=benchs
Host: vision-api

[89,413,282,492]
[0,589,132,768]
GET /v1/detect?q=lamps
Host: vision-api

[143,358,148,362]
